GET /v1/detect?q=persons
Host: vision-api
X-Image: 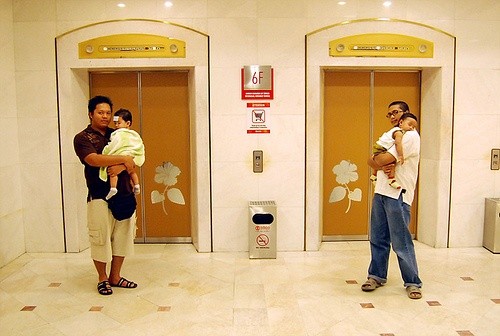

[105,108,146,200]
[369,112,418,190]
[361,100,423,300]
[73,96,137,296]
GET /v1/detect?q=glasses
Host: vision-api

[386,110,405,118]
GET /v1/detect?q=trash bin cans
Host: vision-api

[248,200,277,259]
[482,197,500,254]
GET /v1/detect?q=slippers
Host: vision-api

[109,277,137,288]
[406,284,422,299]
[97,281,113,295]
[361,277,385,291]
